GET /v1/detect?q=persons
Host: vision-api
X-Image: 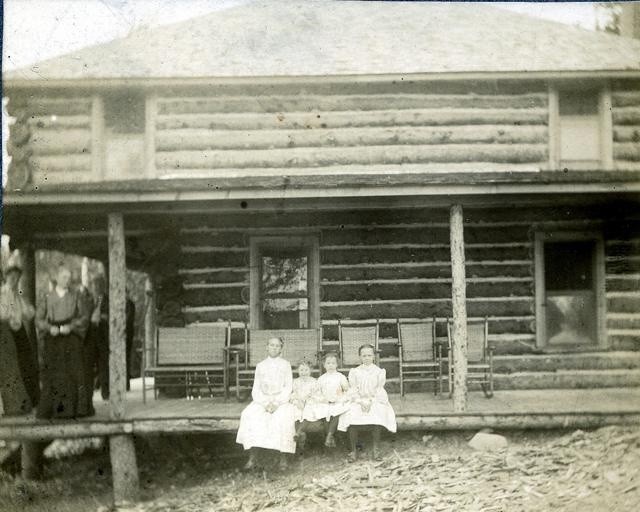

[313,354,351,448]
[1,256,134,418]
[289,360,318,447]
[336,343,397,462]
[235,337,296,472]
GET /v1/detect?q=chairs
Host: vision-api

[336,318,496,399]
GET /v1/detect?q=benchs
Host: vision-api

[139,323,324,400]
